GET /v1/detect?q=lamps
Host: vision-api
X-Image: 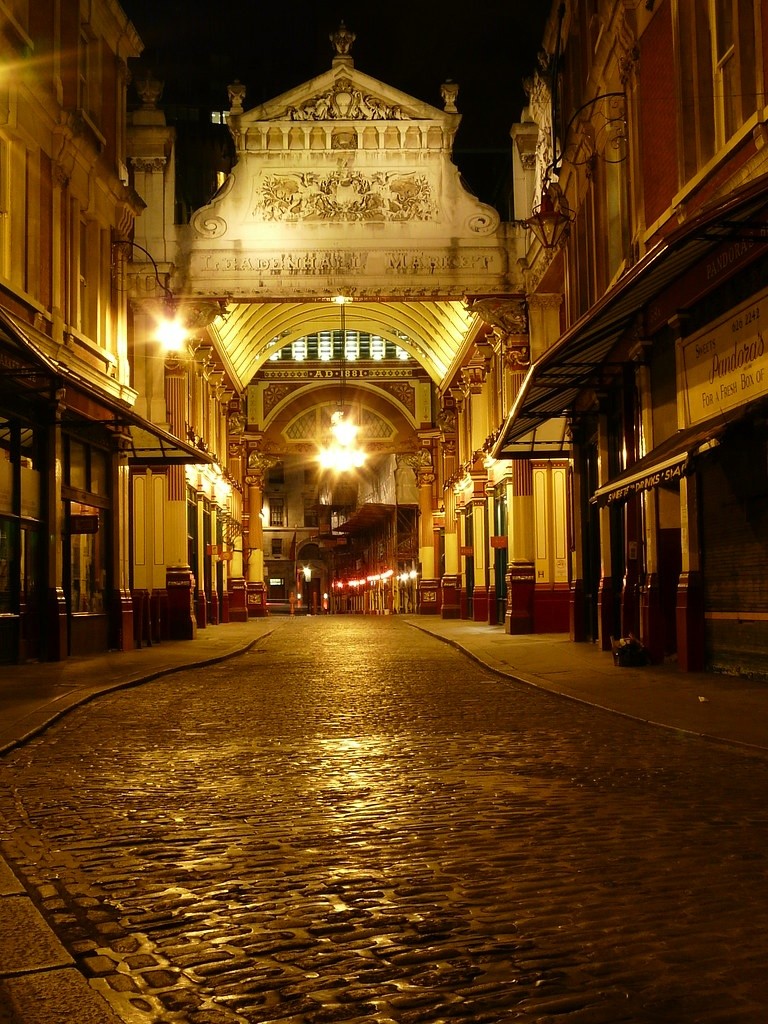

[442,423,504,492]
[186,423,244,494]
[523,92,625,249]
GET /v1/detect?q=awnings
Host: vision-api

[333,503,395,533]
[0,308,213,462]
[489,172,767,458]
[595,395,768,509]
